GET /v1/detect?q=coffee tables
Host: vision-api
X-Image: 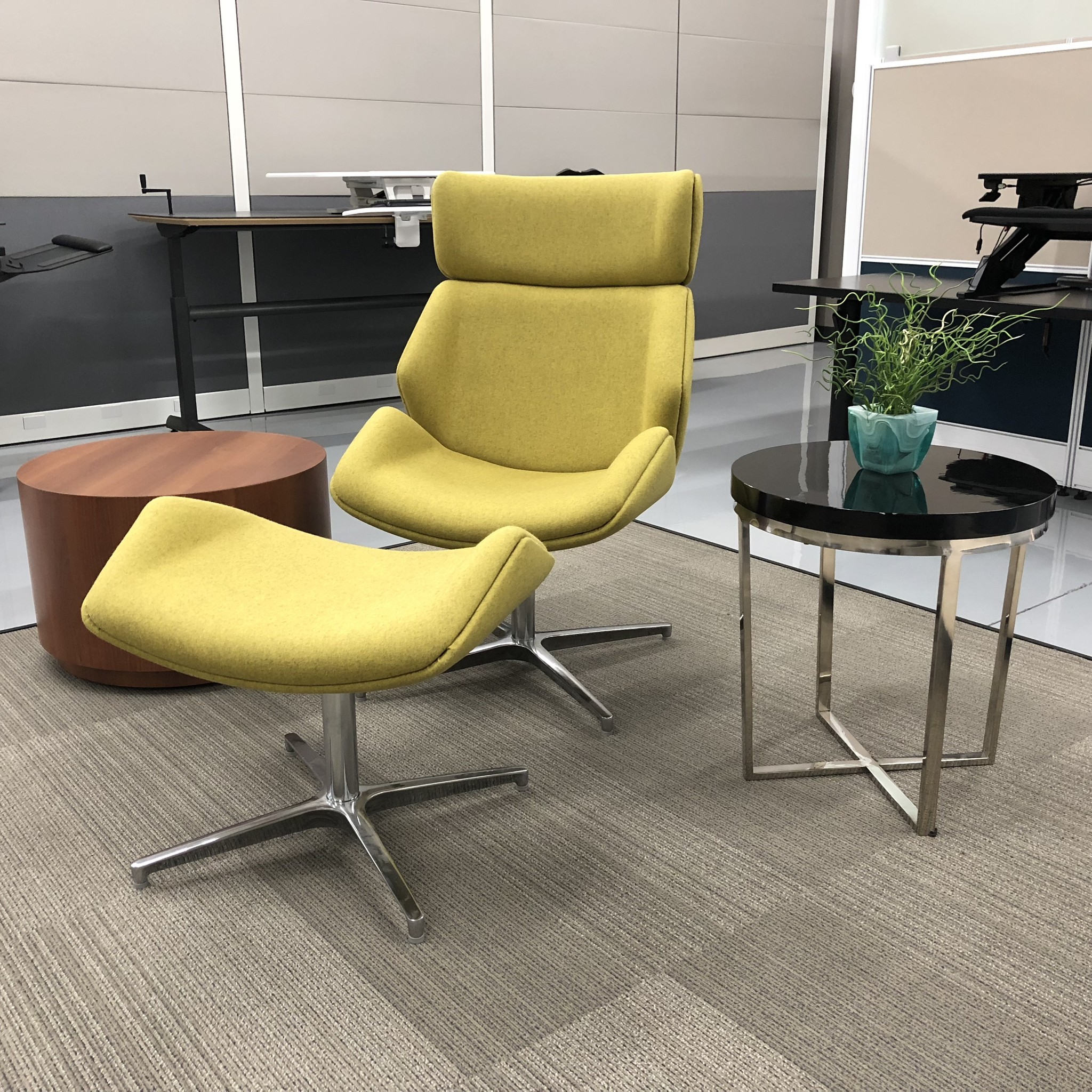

[17,431,331,688]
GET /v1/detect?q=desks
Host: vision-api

[772,273,1092,320]
[127,214,437,432]
[731,441,1059,836]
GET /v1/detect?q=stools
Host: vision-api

[82,495,555,944]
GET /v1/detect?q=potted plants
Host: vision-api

[782,259,1070,474]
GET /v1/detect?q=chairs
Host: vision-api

[329,169,705,732]
